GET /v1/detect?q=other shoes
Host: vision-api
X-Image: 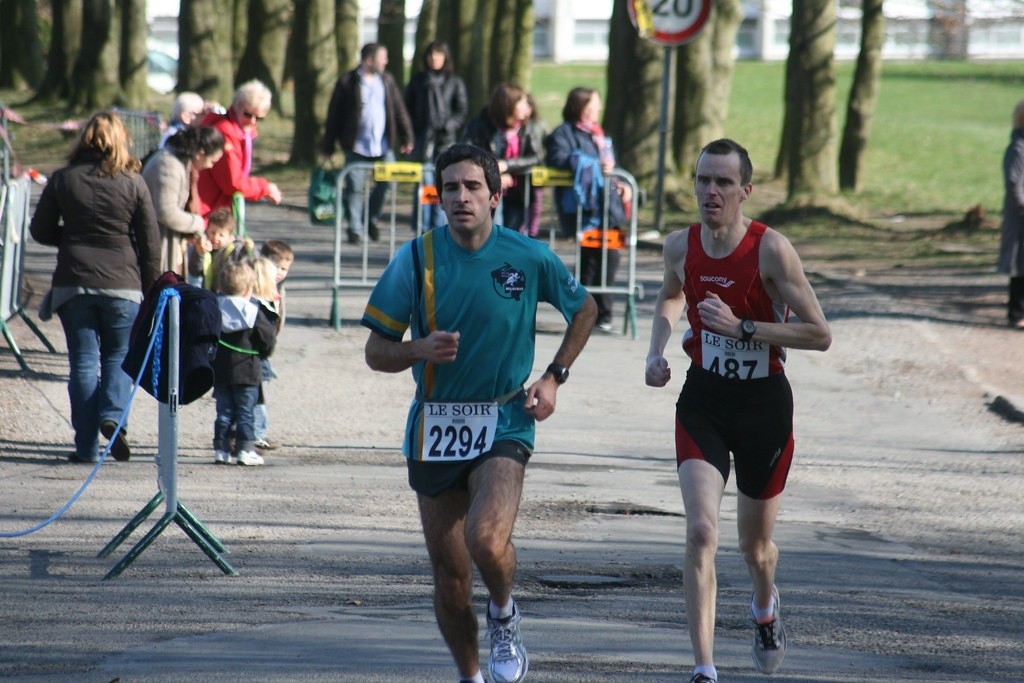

[101,421,131,461]
[1010,320,1024,328]
[69,452,98,463]
[348,231,364,245]
[368,223,380,240]
[596,323,622,334]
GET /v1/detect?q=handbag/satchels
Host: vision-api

[310,154,343,227]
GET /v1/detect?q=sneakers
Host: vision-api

[238,450,264,465]
[485,597,529,682]
[749,583,787,673]
[214,449,237,465]
[689,673,717,683]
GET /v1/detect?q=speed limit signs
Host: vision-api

[627,0,712,44]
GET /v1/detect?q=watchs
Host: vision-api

[739,318,756,341]
[546,363,570,385]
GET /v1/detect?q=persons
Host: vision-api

[29,112,162,464]
[542,87,626,333]
[646,138,832,683]
[230,240,294,456]
[159,92,204,148]
[212,260,276,465]
[996,99,1024,330]
[404,42,469,233]
[519,93,547,236]
[322,43,414,243]
[187,209,259,296]
[141,125,225,284]
[198,78,283,231]
[359,142,597,683]
[465,84,543,229]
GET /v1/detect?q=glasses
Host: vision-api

[244,111,263,121]
